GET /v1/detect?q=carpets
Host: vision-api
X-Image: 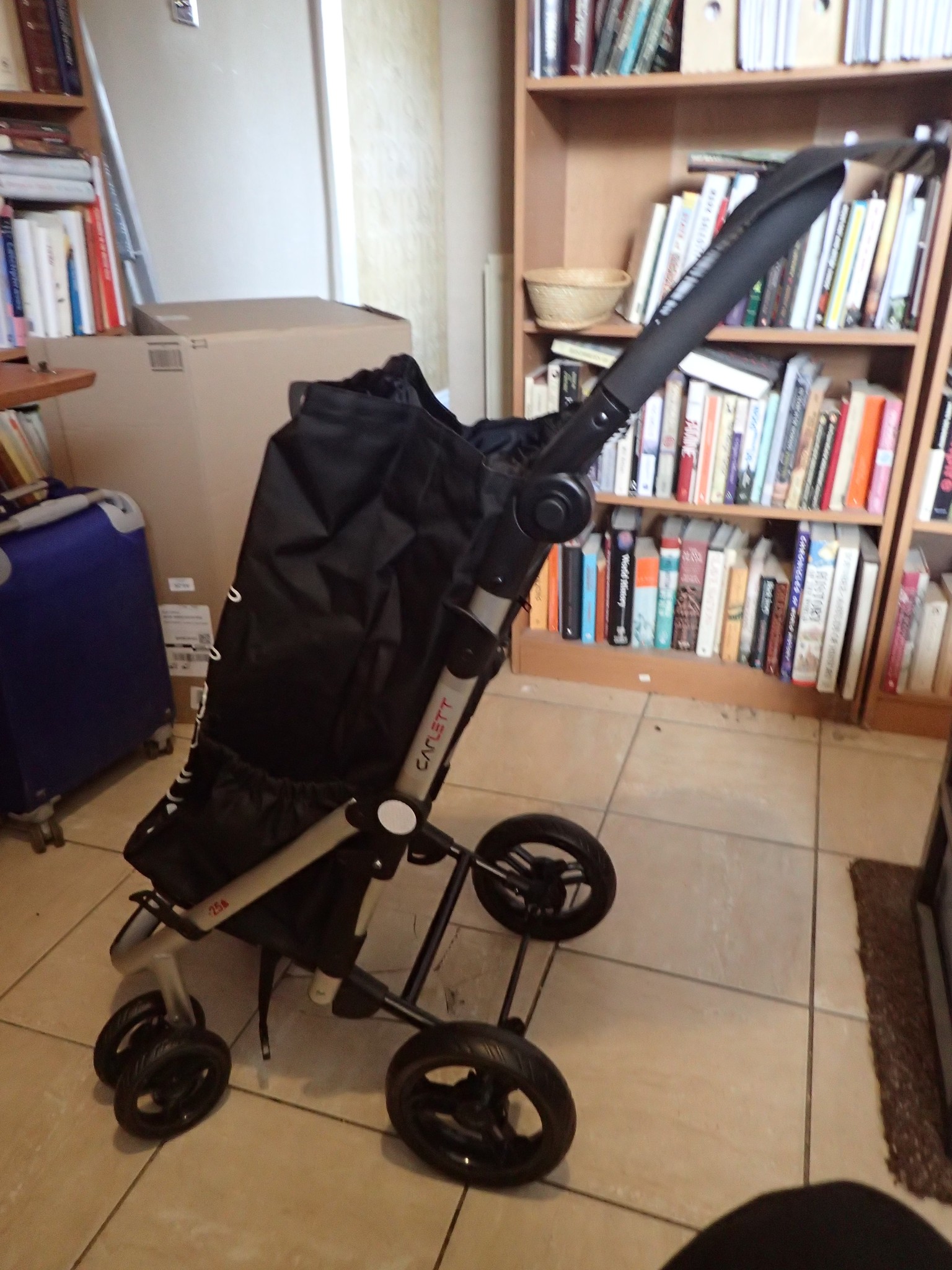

[850,855,952,1206]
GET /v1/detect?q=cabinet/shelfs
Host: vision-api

[511,0,952,740]
[0,0,132,363]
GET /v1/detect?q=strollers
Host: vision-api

[91,136,952,1193]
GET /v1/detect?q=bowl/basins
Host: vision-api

[522,267,632,332]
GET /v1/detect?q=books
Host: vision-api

[884,545,952,706]
[0,116,141,351]
[0,0,83,96]
[511,492,878,704]
[624,140,941,333]
[524,337,952,529]
[530,0,952,80]
[0,404,56,515]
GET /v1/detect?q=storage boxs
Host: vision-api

[24,298,415,724]
